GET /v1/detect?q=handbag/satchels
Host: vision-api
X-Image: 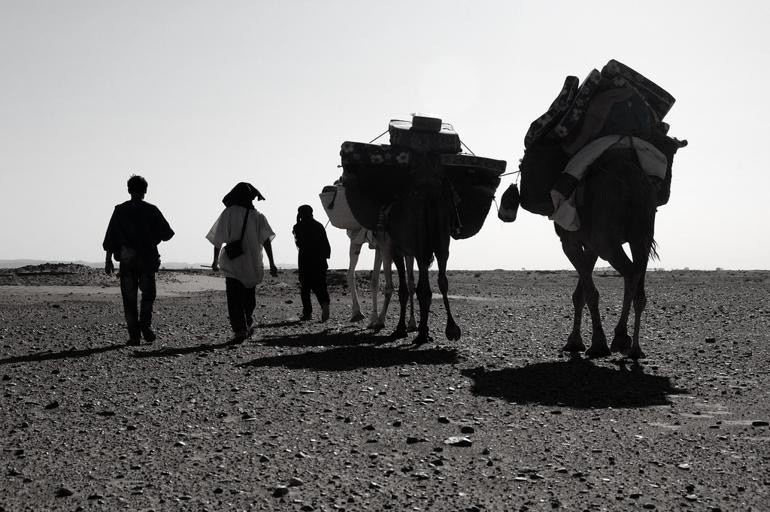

[224,239,245,258]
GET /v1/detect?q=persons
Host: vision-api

[292,204,330,321]
[205,181,278,343]
[102,176,175,346]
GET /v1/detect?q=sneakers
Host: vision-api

[123,321,157,345]
[299,313,315,320]
[320,303,330,322]
[228,326,255,344]
